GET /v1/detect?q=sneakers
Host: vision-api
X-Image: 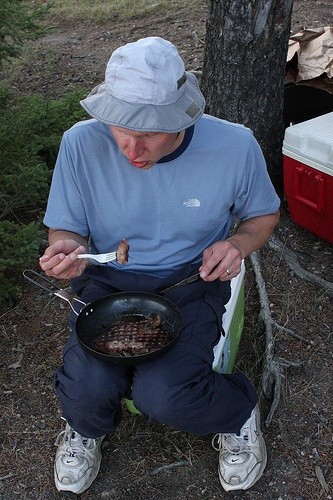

[211,403,268,493]
[54,422,104,495]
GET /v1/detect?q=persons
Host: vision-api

[38,37,282,498]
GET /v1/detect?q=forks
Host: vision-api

[39,251,117,263]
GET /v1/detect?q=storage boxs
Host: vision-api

[282,111,333,244]
[122,257,247,416]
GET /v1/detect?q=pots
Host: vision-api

[23,269,184,362]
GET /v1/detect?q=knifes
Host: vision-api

[160,272,199,295]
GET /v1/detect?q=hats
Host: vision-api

[78,37,205,133]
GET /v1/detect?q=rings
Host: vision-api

[226,270,231,275]
